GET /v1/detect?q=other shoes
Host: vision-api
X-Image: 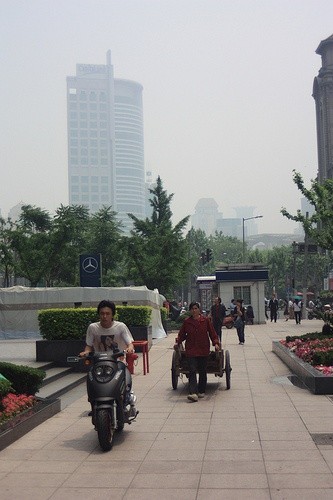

[238,340,245,345]
[187,394,198,402]
[198,392,206,398]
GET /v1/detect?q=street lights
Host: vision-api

[242,215,262,264]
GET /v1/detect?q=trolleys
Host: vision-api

[172,340,232,390]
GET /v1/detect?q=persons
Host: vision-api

[163,294,317,346]
[80,300,138,413]
[177,302,220,402]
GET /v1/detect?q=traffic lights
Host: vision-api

[207,248,213,262]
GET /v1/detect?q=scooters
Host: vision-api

[221,309,236,328]
[73,350,140,451]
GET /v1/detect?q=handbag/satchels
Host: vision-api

[233,318,245,329]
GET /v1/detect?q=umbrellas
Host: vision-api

[287,290,314,300]
